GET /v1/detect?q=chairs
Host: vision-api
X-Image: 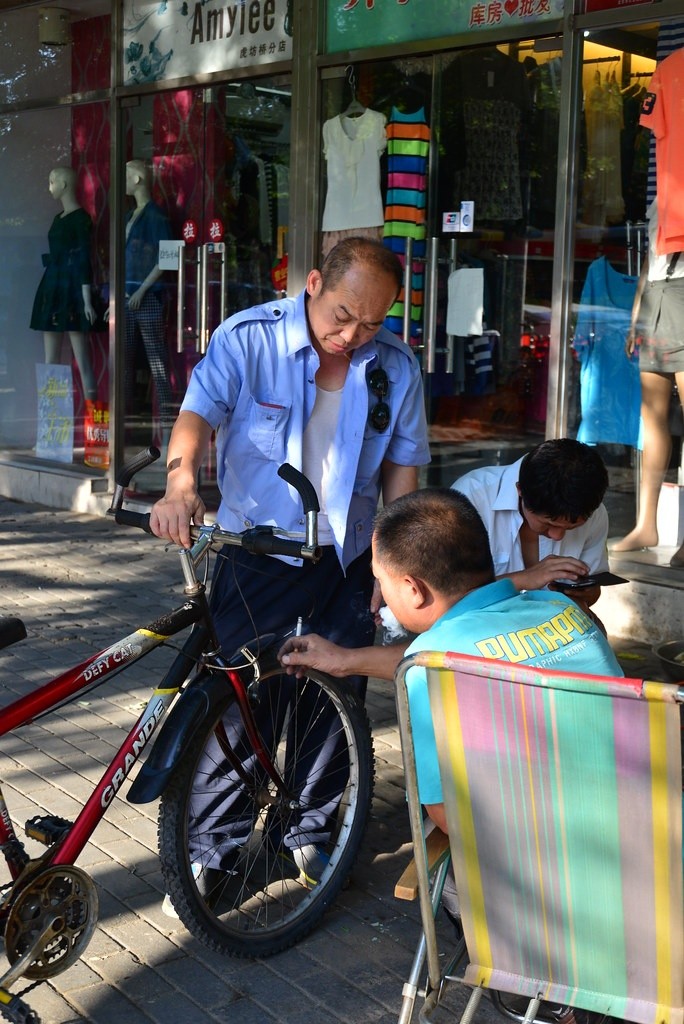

[396,652,684,1024]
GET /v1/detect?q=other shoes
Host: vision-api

[163,861,222,920]
[283,841,330,883]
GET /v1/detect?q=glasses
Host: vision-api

[368,366,390,435]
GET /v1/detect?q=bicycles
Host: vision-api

[0,444,378,1024]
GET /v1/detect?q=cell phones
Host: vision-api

[550,577,596,590]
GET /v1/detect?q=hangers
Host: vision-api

[320,40,684,139]
[218,121,289,178]
[587,235,656,273]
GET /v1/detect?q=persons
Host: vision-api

[29,167,107,451]
[276,487,625,1024]
[378,437,611,639]
[612,196,684,567]
[149,237,431,919]
[104,159,174,466]
